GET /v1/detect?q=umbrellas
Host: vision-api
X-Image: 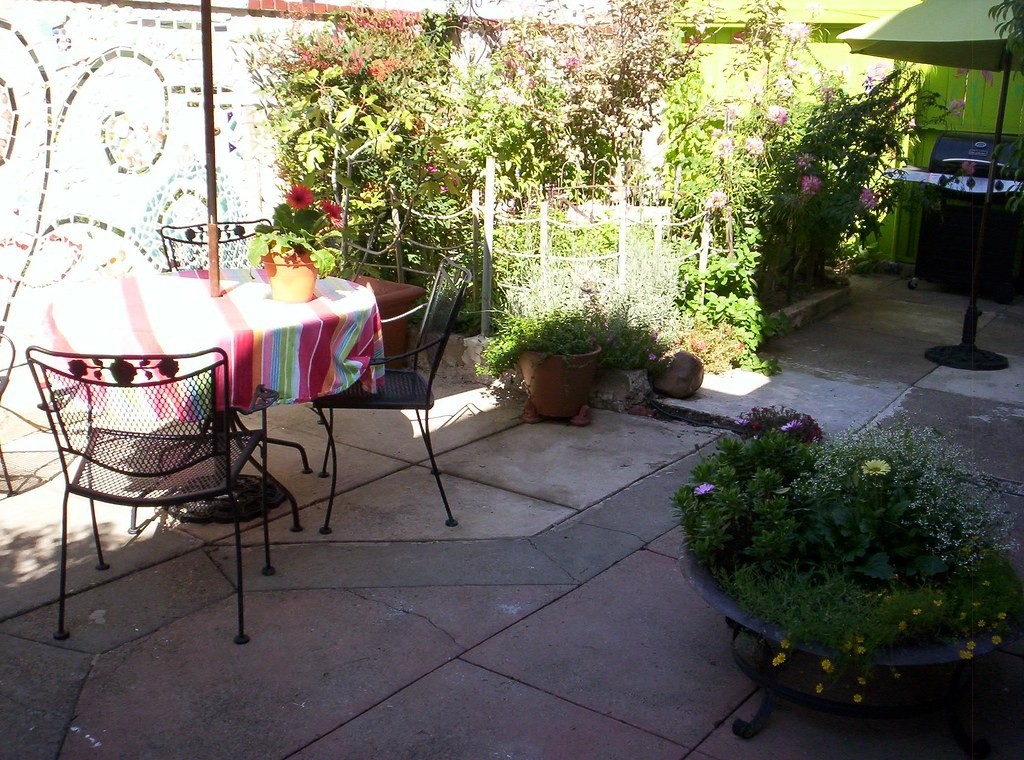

[836,0,1024,345]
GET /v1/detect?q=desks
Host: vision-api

[9,270,386,526]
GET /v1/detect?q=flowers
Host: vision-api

[247,184,348,280]
[473,284,674,399]
[670,402,1024,703]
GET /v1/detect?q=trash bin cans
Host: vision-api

[915,131,1024,300]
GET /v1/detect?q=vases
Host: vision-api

[520,347,645,426]
[267,248,321,302]
[676,539,1024,760]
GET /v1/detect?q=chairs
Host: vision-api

[25,344,276,646]
[161,219,326,429]
[315,258,472,536]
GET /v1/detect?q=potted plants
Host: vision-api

[255,8,479,370]
